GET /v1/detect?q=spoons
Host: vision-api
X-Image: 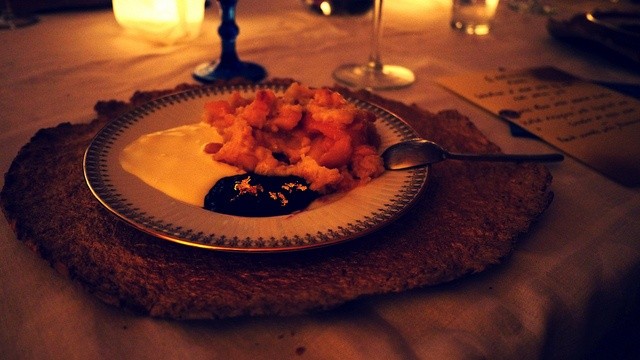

[381,138,566,172]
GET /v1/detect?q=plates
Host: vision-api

[586,5,639,43]
[82,80,430,255]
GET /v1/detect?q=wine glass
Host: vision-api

[190,0,268,86]
[333,0,417,90]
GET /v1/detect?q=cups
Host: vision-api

[450,0,500,38]
